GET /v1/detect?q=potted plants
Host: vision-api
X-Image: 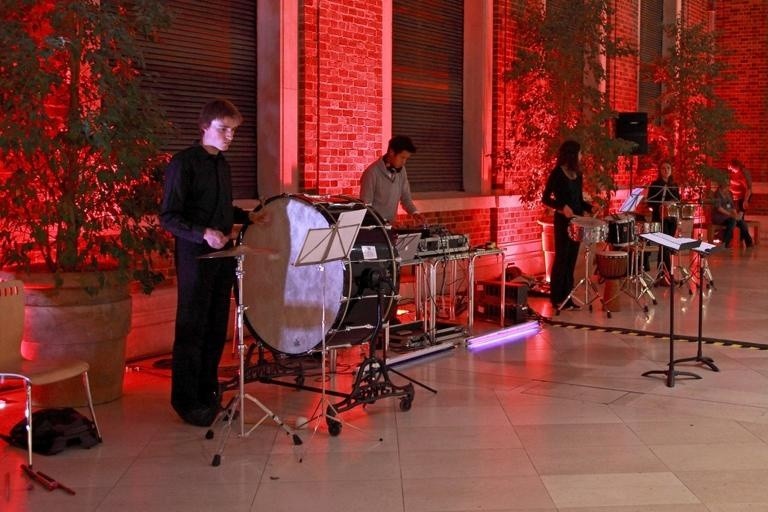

[500,0,644,290]
[0,1,180,408]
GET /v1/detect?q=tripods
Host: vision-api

[640,250,703,387]
[205,259,302,467]
[667,259,720,372]
[605,204,718,320]
[351,322,437,408]
[555,250,612,319]
[287,265,383,464]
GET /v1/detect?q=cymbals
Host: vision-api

[196,243,278,258]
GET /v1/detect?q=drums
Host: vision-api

[668,204,694,219]
[233,192,401,357]
[596,251,629,312]
[567,217,608,243]
[635,223,661,251]
[603,213,636,246]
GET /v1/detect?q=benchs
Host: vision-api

[692,220,760,245]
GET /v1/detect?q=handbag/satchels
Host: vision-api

[8,406,98,455]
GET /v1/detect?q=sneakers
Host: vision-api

[552,302,582,311]
[181,405,239,426]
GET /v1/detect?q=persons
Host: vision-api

[360,135,427,349]
[728,160,752,219]
[648,162,680,271]
[713,177,760,248]
[158,99,271,427]
[542,140,602,311]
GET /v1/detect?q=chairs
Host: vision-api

[0,279,103,472]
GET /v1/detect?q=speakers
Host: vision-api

[614,111,649,156]
[476,279,528,323]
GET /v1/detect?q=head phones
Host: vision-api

[382,153,402,174]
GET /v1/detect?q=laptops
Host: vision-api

[395,233,423,260]
